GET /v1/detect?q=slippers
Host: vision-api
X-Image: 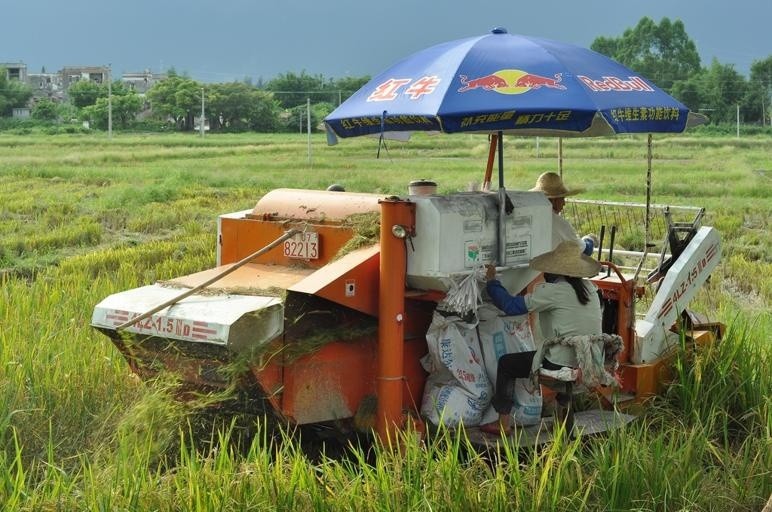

[481,423,511,436]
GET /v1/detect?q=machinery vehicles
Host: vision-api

[88,181,732,467]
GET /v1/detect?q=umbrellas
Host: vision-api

[321,25,691,270]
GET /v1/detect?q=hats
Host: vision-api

[528,172,582,199]
[530,241,602,278]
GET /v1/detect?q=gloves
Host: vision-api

[582,233,599,247]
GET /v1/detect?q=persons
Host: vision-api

[478,239,603,440]
[526,170,599,259]
